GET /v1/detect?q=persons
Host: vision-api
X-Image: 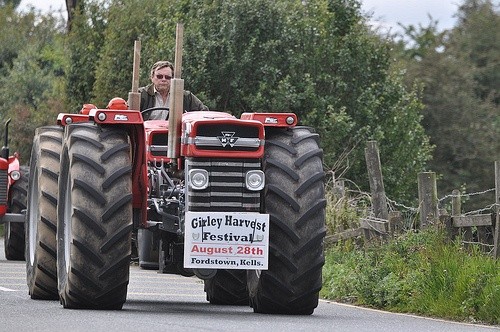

[125,62,208,120]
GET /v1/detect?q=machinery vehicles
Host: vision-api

[25,22,325,315]
[0,118,31,261]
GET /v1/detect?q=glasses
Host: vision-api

[154,74,172,80]
